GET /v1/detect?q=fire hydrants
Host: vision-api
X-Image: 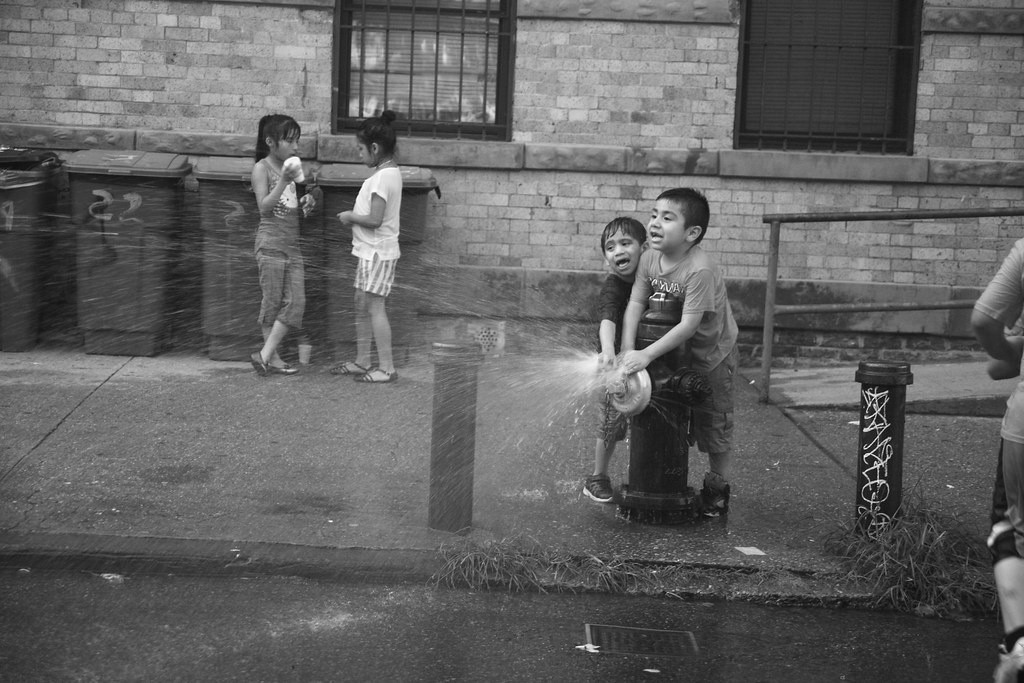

[597,282,702,528]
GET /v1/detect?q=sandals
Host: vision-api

[353,367,399,383]
[330,360,373,375]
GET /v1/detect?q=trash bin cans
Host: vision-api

[0,146,64,353]
[191,156,313,363]
[60,147,194,359]
[314,161,441,370]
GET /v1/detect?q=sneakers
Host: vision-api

[987,629,1024,682]
[582,473,614,504]
[266,358,297,374]
[695,471,731,517]
[250,354,270,378]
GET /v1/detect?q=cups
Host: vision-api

[285,156,306,184]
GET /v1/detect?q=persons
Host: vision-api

[584,215,654,503]
[971,236,1024,682]
[614,186,740,522]
[250,114,317,378]
[330,109,399,384]
